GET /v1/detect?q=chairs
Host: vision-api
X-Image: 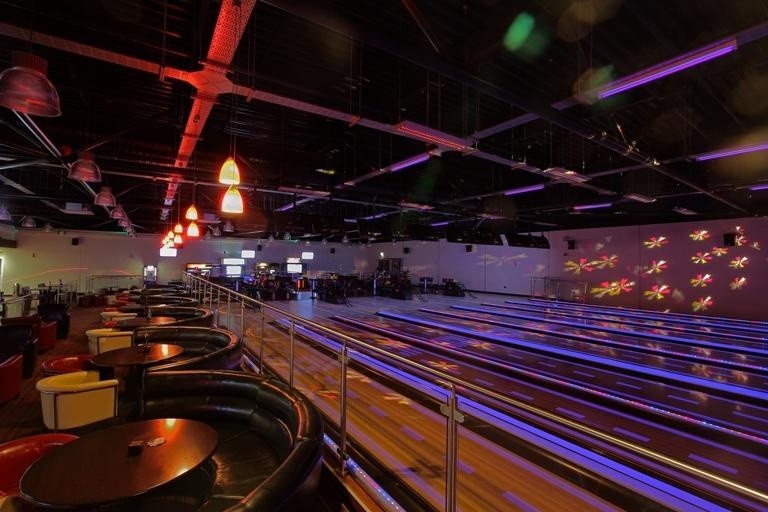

[0,283,324,512]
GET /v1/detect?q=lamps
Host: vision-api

[696,144,767,161]
[597,37,739,104]
[273,197,312,215]
[158,150,248,248]
[365,213,387,221]
[431,222,449,227]
[751,184,767,194]
[574,203,612,212]
[0,1,140,248]
[503,183,547,200]
[388,151,431,176]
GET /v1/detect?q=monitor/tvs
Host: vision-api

[241,250,256,259]
[302,252,313,260]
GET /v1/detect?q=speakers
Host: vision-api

[72,238,79,246]
[258,245,262,251]
[723,233,739,246]
[568,240,577,250]
[404,247,410,253]
[465,245,472,253]
[330,248,335,254]
